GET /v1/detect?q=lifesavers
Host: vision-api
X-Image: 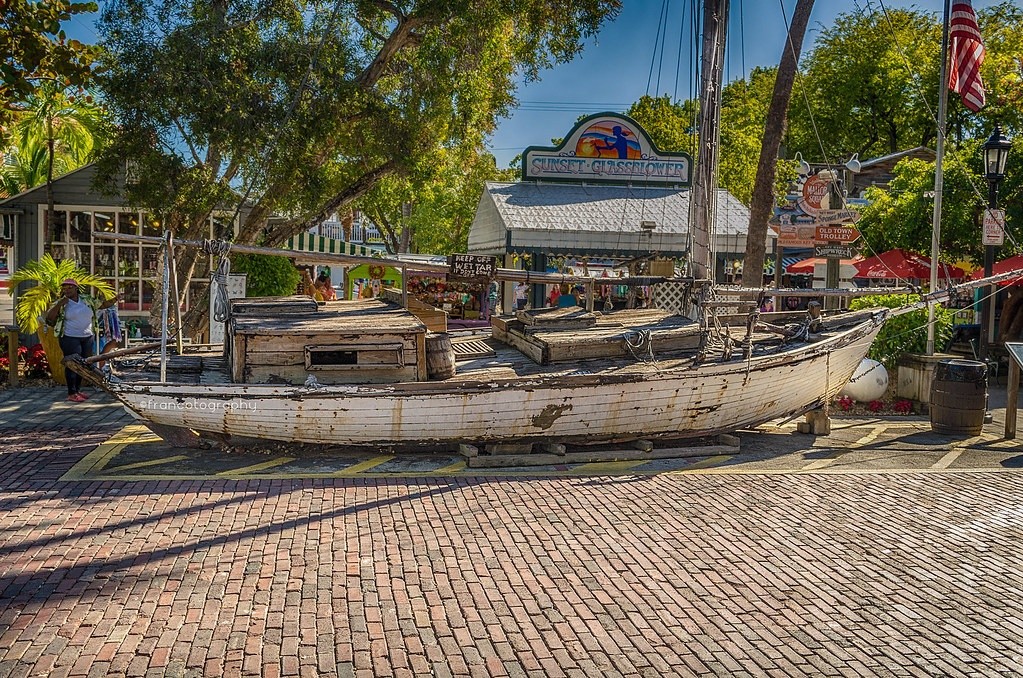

[369,263,385,280]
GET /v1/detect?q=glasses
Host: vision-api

[63,286,73,289]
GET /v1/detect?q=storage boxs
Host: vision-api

[650,259,674,277]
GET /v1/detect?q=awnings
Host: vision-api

[283,231,382,257]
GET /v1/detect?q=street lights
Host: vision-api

[979,118,1012,424]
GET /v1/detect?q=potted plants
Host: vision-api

[5,252,119,386]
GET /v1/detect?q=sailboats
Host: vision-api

[61,0,893,450]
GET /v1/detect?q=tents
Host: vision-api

[852,249,964,287]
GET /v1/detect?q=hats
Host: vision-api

[60,278,77,286]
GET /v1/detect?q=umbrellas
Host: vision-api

[970,256,1023,286]
[785,258,858,274]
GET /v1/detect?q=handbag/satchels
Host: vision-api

[54,306,64,338]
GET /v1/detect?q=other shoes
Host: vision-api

[78,392,89,399]
[68,395,84,402]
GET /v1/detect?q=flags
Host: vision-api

[947,0,987,112]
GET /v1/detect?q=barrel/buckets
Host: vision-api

[928,359,988,436]
[425,331,456,380]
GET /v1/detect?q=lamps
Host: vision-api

[641,221,656,236]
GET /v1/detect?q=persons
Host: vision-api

[306,271,337,302]
[513,281,530,310]
[547,283,586,308]
[759,281,803,312]
[45,279,125,402]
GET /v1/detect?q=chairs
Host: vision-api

[968,338,999,389]
